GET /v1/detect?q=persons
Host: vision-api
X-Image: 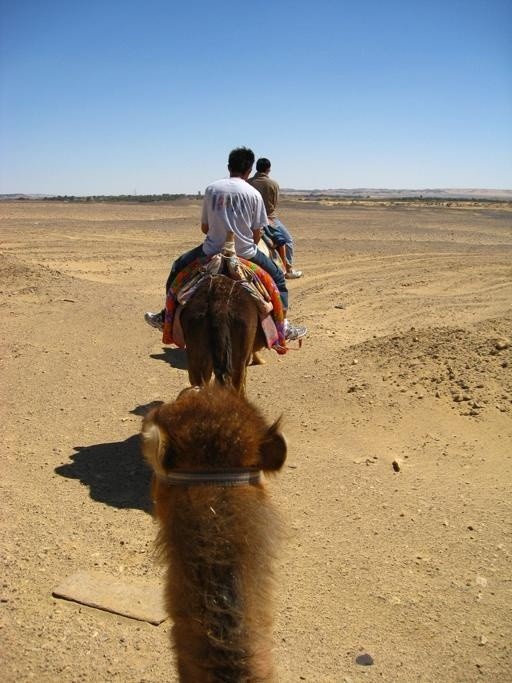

[144,146,306,341]
[246,158,301,278]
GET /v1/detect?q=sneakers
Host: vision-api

[280,322,308,341]
[284,267,304,279]
[145,310,165,331]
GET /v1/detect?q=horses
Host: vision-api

[179,232,273,400]
